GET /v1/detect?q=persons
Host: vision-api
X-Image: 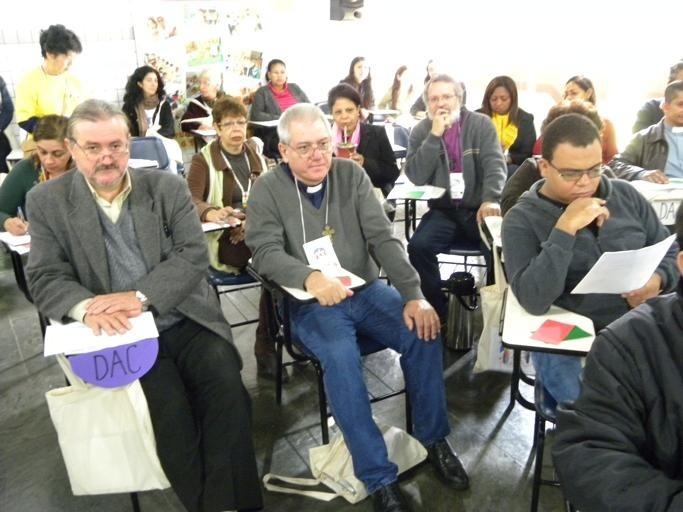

[402,58,535,326]
[499,58,683,401]
[552,202,682,512]
[21,98,266,512]
[325,56,415,219]
[243,103,474,512]
[250,58,307,121]
[0,23,82,305]
[120,65,269,280]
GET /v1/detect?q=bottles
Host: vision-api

[438,271,479,352]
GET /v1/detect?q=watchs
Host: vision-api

[134,290,149,311]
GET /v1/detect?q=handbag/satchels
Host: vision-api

[44,357,172,497]
[145,101,183,175]
[472,242,514,374]
[262,419,429,505]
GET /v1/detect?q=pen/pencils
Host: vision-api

[17,206,25,224]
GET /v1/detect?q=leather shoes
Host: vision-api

[426,438,468,490]
[374,480,412,512]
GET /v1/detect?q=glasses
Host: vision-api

[549,162,604,182]
[217,118,248,127]
[69,133,129,159]
[283,140,334,156]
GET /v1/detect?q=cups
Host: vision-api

[336,143,356,158]
